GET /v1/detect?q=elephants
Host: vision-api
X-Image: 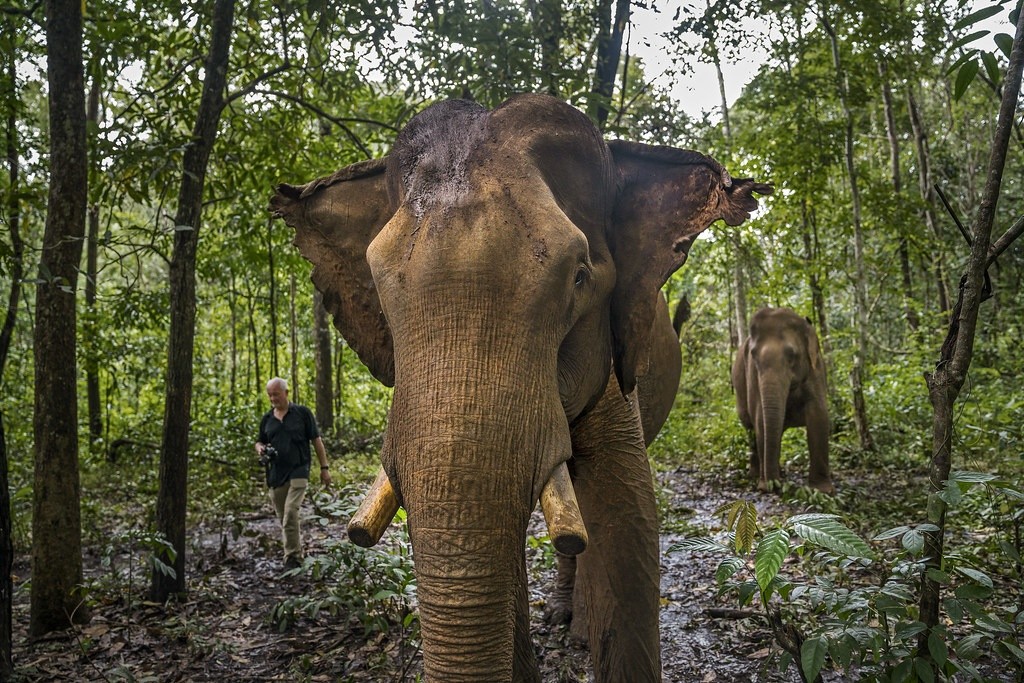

[269,93,776,683]
[731,306,838,497]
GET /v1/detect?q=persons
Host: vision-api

[254,377,332,569]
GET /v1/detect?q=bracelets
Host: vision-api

[320,466,329,470]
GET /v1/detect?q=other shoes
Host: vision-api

[284,553,304,568]
